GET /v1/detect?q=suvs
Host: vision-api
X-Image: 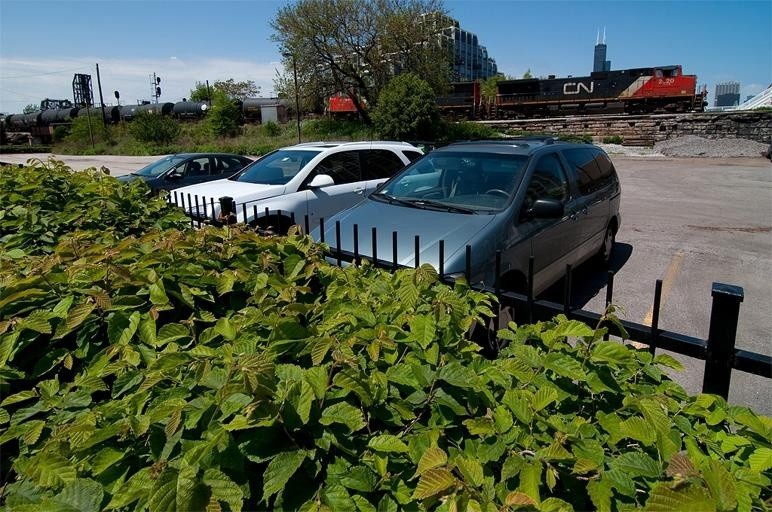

[160,140,442,238]
[304,141,622,359]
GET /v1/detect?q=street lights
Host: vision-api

[283,53,302,146]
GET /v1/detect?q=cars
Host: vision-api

[115,152,284,201]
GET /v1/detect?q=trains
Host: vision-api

[0,64,709,136]
